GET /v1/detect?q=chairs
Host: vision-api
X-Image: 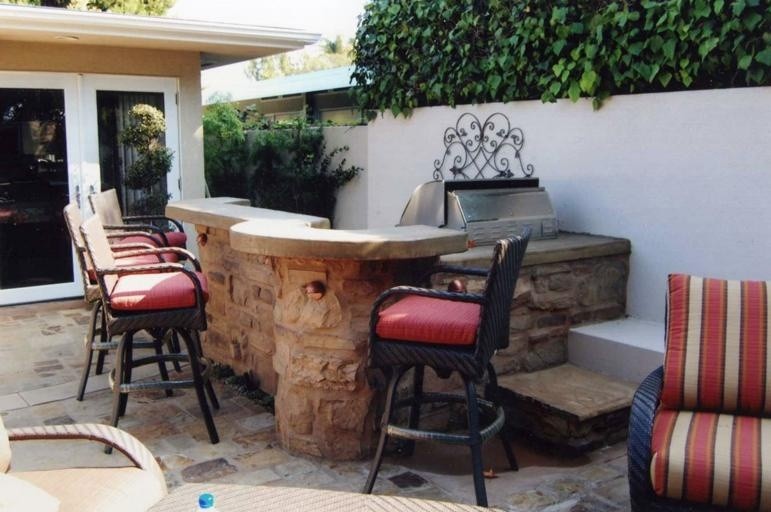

[628,275,768,509]
[360,227,531,507]
[0,413,165,512]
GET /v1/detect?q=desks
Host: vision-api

[147,482,508,512]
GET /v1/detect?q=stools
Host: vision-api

[63,201,184,402]
[79,213,218,455]
[88,188,188,262]
